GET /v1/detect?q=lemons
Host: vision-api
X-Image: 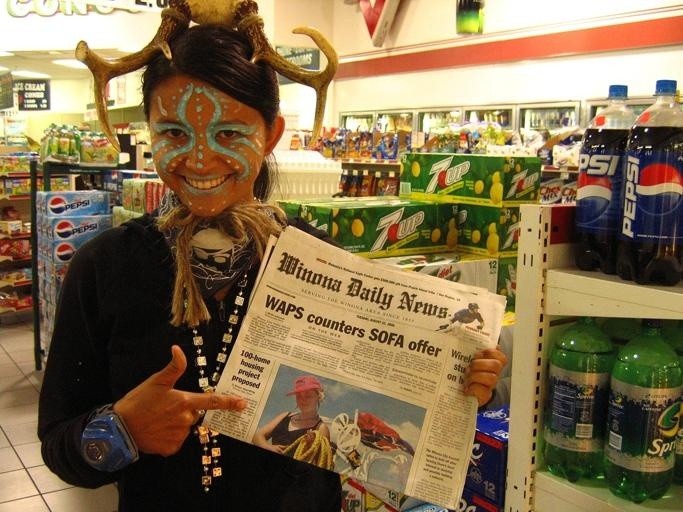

[500,289,506,296]
[308,161,521,256]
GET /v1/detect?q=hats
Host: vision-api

[285,376,323,396]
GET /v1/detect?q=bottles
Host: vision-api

[524,78,682,289]
[39,122,81,165]
[541,318,683,503]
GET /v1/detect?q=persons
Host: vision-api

[436,302,484,336]
[250,374,334,471]
[31,20,509,509]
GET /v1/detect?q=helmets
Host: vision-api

[468,303,480,310]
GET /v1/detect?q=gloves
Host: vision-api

[440,324,449,329]
[477,325,483,330]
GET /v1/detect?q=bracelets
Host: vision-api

[79,402,139,473]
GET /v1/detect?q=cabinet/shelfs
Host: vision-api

[503,202,682,512]
[0,136,125,373]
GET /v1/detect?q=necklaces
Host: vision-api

[180,262,248,493]
